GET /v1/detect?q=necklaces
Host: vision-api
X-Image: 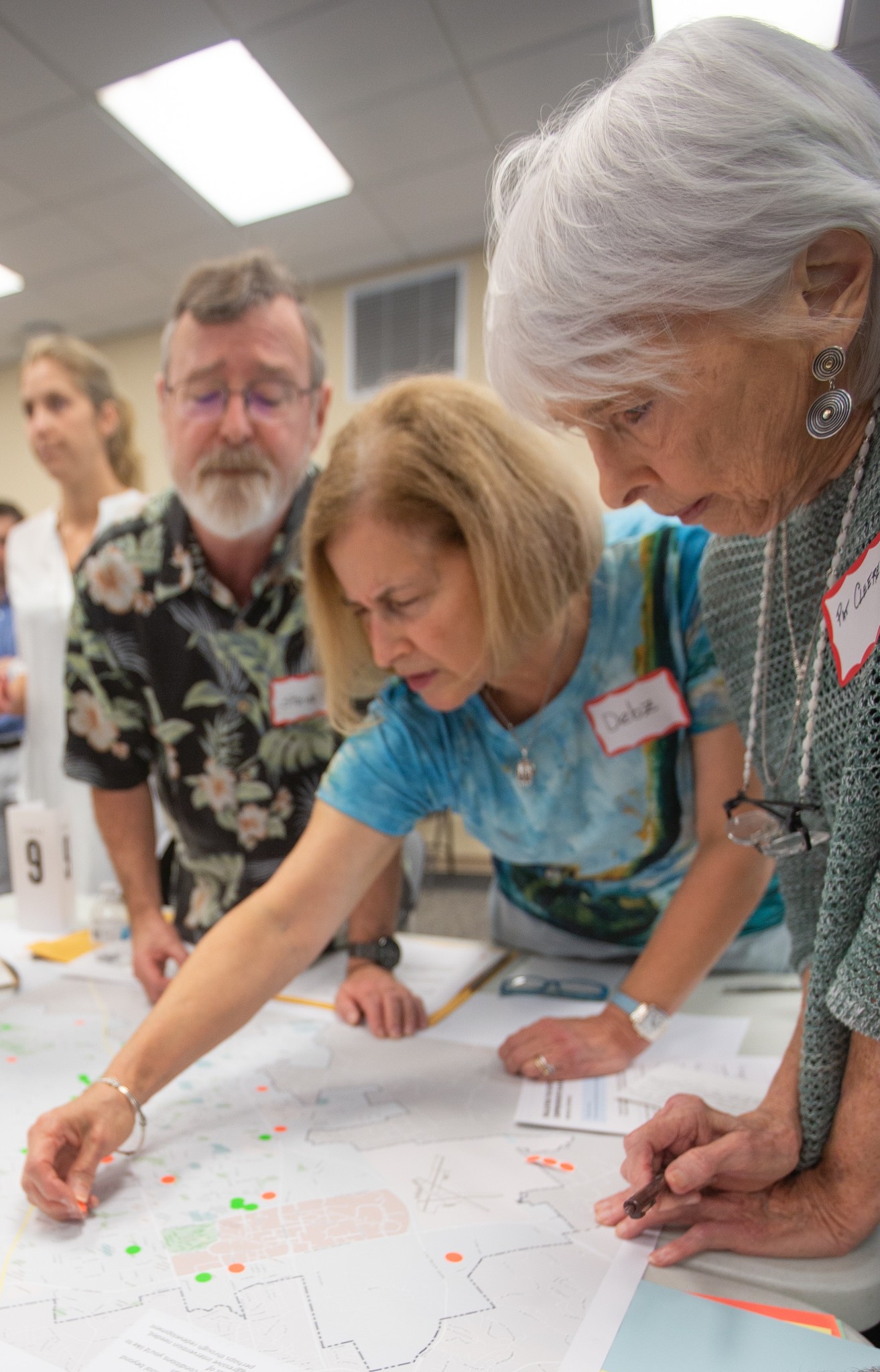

[758,520,837,789]
[481,606,569,789]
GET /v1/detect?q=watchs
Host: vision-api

[349,937,401,969]
[610,987,674,1047]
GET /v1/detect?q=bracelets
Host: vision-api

[93,1075,147,1156]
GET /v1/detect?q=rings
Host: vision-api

[534,1055,556,1077]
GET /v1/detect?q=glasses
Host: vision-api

[165,375,318,419]
[721,792,832,860]
[499,974,608,1000]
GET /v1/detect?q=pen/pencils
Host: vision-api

[623,1174,669,1218]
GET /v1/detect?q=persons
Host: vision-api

[61,257,434,1039]
[0,335,174,894]
[20,373,791,1224]
[481,18,880,1274]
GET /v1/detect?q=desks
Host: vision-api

[1,890,880,1372]
